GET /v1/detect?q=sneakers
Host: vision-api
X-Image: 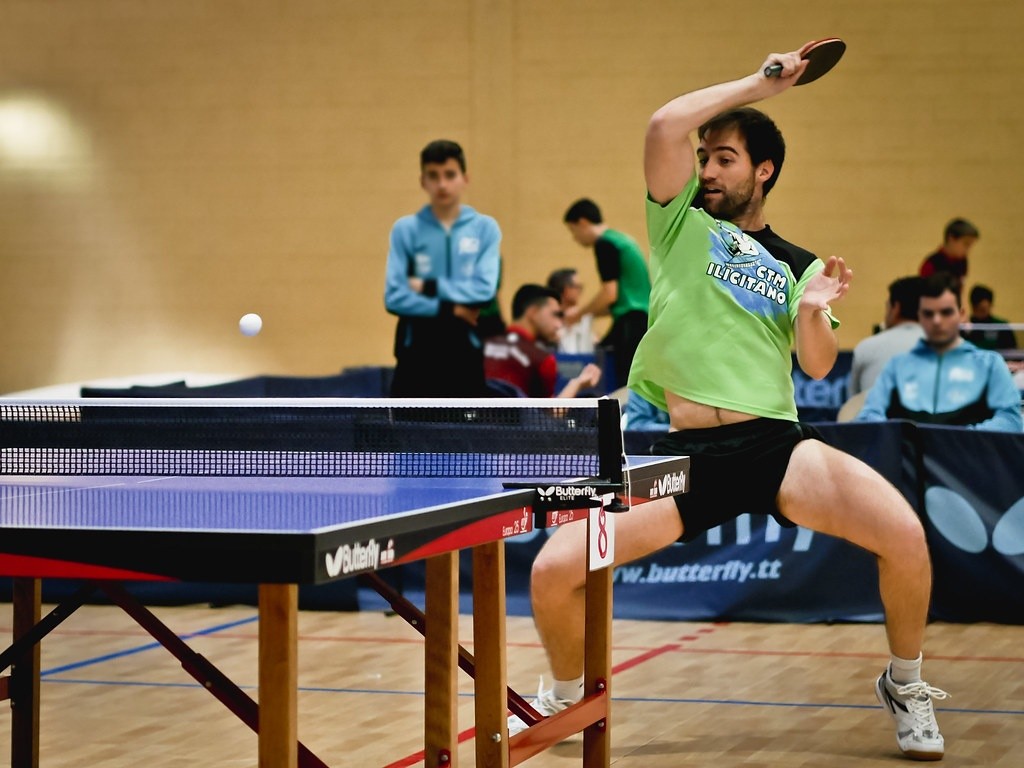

[506,675,576,738]
[874,660,951,761]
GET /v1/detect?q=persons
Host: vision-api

[508,39,963,761]
[381,139,503,422]
[853,271,1024,436]
[852,217,1024,394]
[484,269,601,419]
[561,197,650,398]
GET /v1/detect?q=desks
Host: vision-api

[0,446,691,768]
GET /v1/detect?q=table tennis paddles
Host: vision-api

[763,37,847,87]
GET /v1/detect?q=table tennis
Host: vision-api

[238,312,263,337]
